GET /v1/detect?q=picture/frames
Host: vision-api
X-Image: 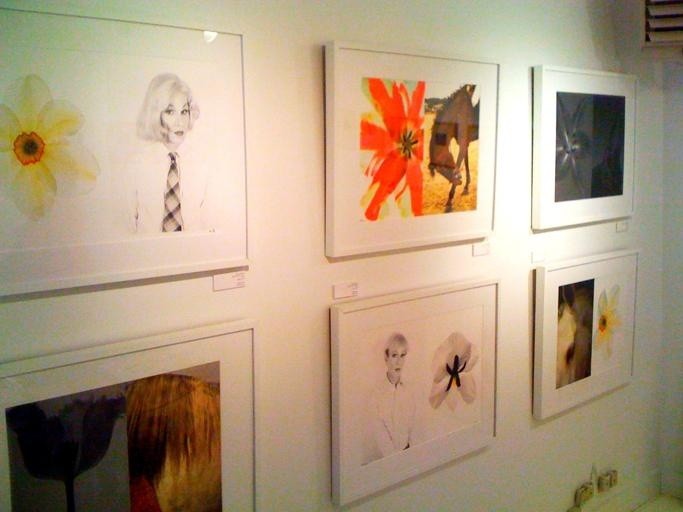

[528,63,644,236]
[1,313,270,512]
[0,3,256,304]
[324,36,503,264]
[528,246,646,423]
[327,274,508,510]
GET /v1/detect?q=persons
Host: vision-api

[108,73,219,245]
[363,329,421,466]
[557,287,600,390]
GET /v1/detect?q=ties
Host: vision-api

[161,151,185,234]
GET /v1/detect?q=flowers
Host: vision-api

[356,74,428,223]
[422,327,481,417]
[592,283,630,364]
[0,69,103,226]
